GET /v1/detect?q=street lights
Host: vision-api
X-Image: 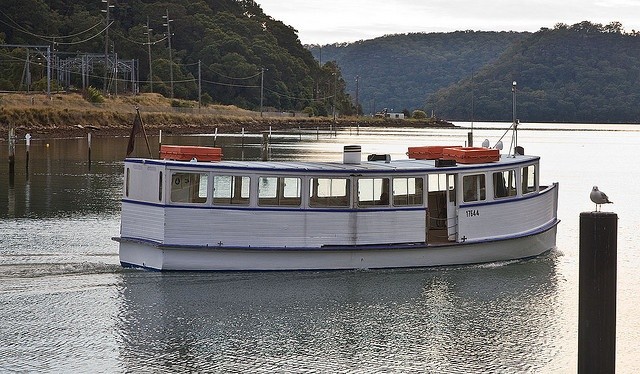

[354,75,361,117]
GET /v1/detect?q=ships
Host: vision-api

[112,80,560,272]
